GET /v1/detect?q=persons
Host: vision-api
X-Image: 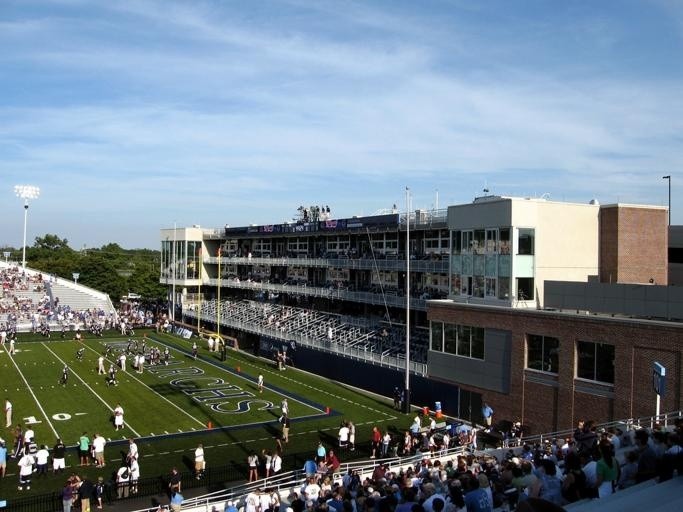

[132,353,139,368]
[140,354,145,374]
[97,356,106,375]
[4,398,12,428]
[114,403,124,431]
[135,353,141,373]
[278,397,289,423]
[481,402,494,426]
[397,387,404,414]
[393,385,401,411]
[256,372,264,394]
[272,349,283,372]
[106,364,118,388]
[57,364,69,388]
[0,201,441,364]
[119,354,126,372]
[406,388,413,415]
[0,412,682,511]
[279,351,289,371]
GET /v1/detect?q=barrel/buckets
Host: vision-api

[422,406,430,416]
[434,401,442,417]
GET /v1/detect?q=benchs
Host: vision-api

[561,477,683,512]
[0,267,114,329]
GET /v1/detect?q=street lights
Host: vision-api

[661,175,671,229]
[72,272,79,284]
[2,251,9,263]
[12,185,39,269]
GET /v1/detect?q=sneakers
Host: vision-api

[114,425,125,432]
[136,371,144,374]
[18,486,31,491]
[96,463,106,468]
[98,372,107,375]
[370,455,375,459]
[97,505,102,508]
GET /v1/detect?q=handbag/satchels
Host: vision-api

[121,470,129,480]
